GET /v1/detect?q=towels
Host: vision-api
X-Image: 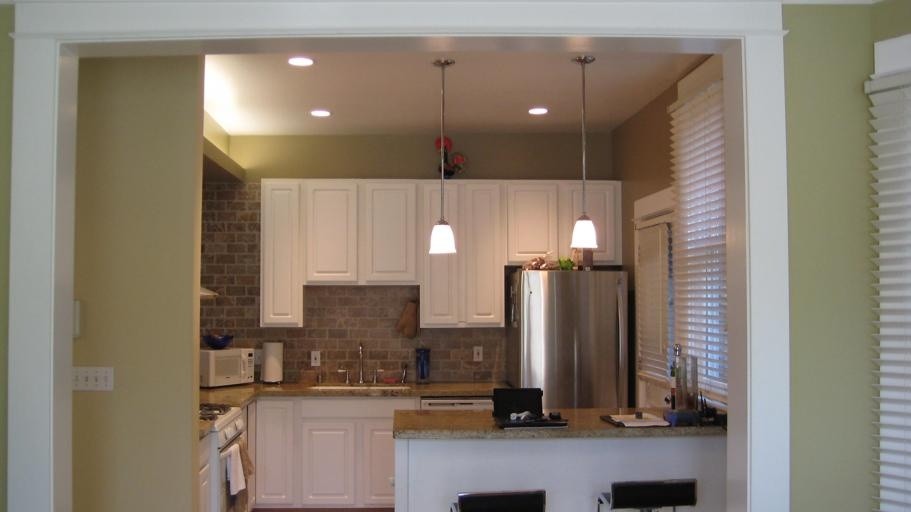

[226,443,247,494]
[233,438,255,512]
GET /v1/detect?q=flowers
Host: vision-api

[432,135,468,178]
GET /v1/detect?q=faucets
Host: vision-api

[358,343,367,384]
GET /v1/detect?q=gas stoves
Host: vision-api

[196,403,242,432]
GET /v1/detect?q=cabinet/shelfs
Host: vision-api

[418,179,507,330]
[247,396,297,509]
[259,179,303,329]
[303,178,419,286]
[199,430,223,511]
[297,397,420,509]
[507,179,623,267]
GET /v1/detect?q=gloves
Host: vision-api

[395,301,417,339]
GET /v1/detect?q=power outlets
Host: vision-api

[72,366,116,392]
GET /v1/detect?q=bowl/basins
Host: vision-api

[200,333,236,351]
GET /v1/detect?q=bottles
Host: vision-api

[669,344,683,410]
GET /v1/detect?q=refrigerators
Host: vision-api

[505,265,630,407]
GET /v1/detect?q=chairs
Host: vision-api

[595,476,699,511]
[447,488,549,512]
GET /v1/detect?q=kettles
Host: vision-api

[414,347,430,386]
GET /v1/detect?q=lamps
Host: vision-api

[427,58,458,255]
[569,55,599,249]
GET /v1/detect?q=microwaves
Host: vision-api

[199,347,254,388]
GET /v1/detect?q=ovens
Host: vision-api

[209,416,247,512]
